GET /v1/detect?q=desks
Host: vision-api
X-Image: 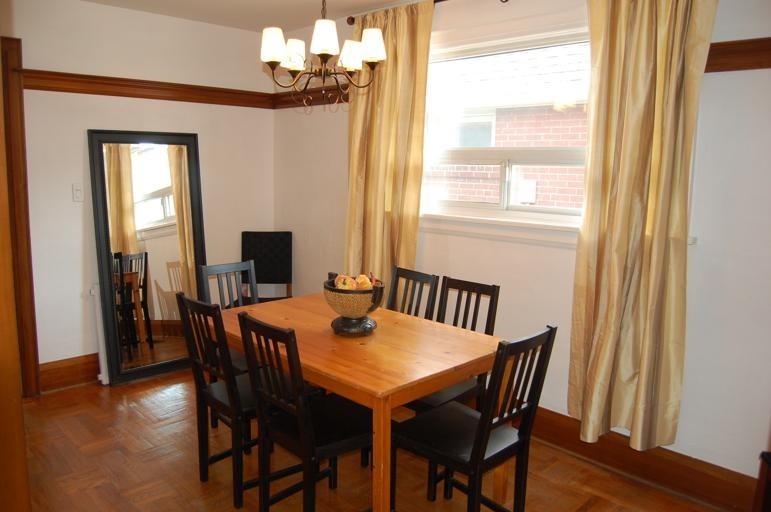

[187,291,512,511]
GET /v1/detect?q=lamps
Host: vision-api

[258,0,387,112]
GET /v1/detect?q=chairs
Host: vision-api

[238,312,371,510]
[175,291,321,509]
[110,250,155,363]
[384,265,499,419]
[392,324,561,511]
[199,259,265,428]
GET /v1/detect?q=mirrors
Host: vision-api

[88,131,200,384]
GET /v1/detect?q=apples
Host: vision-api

[334,274,372,289]
